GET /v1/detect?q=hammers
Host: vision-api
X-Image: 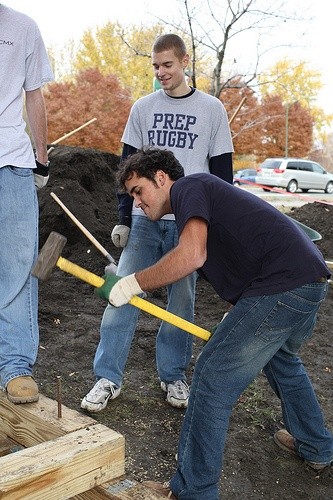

[31,232,211,342]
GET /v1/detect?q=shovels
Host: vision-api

[50,192,118,267]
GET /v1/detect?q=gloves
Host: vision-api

[111,225,131,248]
[94,273,144,308]
[33,159,50,191]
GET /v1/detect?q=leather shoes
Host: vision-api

[273,429,329,469]
[142,480,176,500]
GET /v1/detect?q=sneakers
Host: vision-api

[161,379,192,409]
[81,377,121,412]
[7,375,39,403]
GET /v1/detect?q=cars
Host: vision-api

[254,158,333,195]
[233,168,257,186]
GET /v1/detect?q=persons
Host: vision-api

[96,147,333,500]
[1,3,53,405]
[80,35,235,411]
